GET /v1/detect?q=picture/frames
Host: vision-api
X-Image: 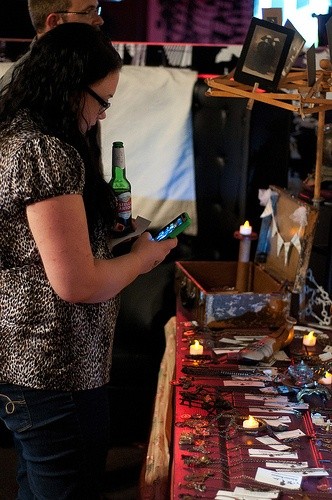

[233,16,295,93]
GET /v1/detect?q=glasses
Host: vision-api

[53,5,102,17]
[86,87,111,116]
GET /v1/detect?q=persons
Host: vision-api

[0,0,103,91]
[0,21,178,500]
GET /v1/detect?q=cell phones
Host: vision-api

[152,210,192,242]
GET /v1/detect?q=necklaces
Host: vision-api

[178,419,303,500]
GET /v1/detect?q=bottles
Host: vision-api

[108,141,133,238]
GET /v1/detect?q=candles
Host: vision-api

[320,371,332,384]
[190,339,203,355]
[243,415,259,433]
[240,220,253,234]
[303,331,316,346]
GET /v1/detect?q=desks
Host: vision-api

[167,314,332,500]
